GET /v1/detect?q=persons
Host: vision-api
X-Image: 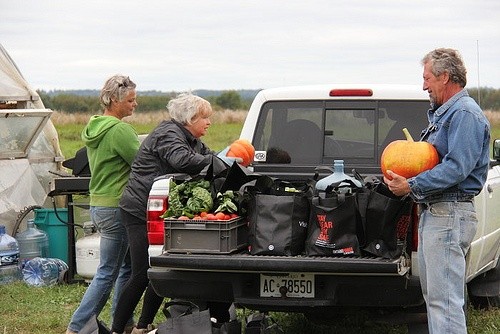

[383,47,491,334]
[111,95,243,334]
[65,74,148,332]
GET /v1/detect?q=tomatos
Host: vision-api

[176,211,238,220]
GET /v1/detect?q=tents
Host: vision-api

[0,42,66,235]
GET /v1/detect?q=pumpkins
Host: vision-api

[226,140,255,167]
[380,128,440,182]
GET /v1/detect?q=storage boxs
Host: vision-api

[163,216,247,255]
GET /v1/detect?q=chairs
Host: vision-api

[276,119,321,163]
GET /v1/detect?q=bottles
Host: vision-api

[314,159,362,205]
[15,219,48,269]
[22,257,69,287]
[0,225,21,285]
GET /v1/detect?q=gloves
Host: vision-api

[217,146,244,168]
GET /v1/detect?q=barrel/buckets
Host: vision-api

[33,208,68,265]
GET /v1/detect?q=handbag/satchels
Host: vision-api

[211,302,242,334]
[247,180,311,256]
[204,154,263,198]
[305,179,359,258]
[155,300,223,334]
[357,180,413,259]
[243,312,285,334]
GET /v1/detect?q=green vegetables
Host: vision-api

[214,190,246,213]
[159,178,213,218]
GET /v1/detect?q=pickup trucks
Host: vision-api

[147,83,500,330]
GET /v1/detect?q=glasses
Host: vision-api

[119,76,130,87]
